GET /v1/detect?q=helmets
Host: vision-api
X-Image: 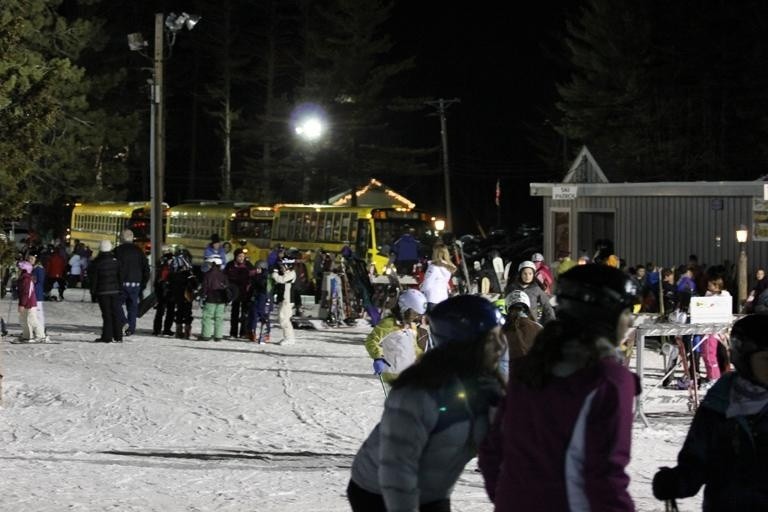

[279,255,295,271]
[398,288,427,317]
[18,261,33,274]
[506,289,531,310]
[728,312,765,380]
[430,294,507,348]
[555,264,642,314]
[532,253,546,262]
[518,261,538,274]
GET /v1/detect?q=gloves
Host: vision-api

[373,359,392,375]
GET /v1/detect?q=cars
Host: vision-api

[0,226,32,254]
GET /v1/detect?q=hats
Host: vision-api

[209,233,223,245]
[233,248,243,257]
[28,250,40,258]
[99,240,113,252]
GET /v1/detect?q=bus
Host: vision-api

[164,198,276,284]
[67,200,170,259]
[268,204,443,302]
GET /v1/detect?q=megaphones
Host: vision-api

[184,13,202,30]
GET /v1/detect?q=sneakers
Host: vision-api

[682,378,718,390]
[278,340,295,347]
[95,329,134,342]
[152,330,258,341]
[18,337,46,343]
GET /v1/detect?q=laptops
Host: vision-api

[690,296,733,326]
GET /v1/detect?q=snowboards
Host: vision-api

[137,292,158,317]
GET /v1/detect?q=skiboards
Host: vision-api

[10,333,61,344]
[329,279,343,324]
[676,333,698,412]
[492,257,512,297]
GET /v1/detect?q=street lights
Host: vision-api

[127,11,203,305]
[733,224,750,312]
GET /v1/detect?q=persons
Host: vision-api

[0,225,768,511]
